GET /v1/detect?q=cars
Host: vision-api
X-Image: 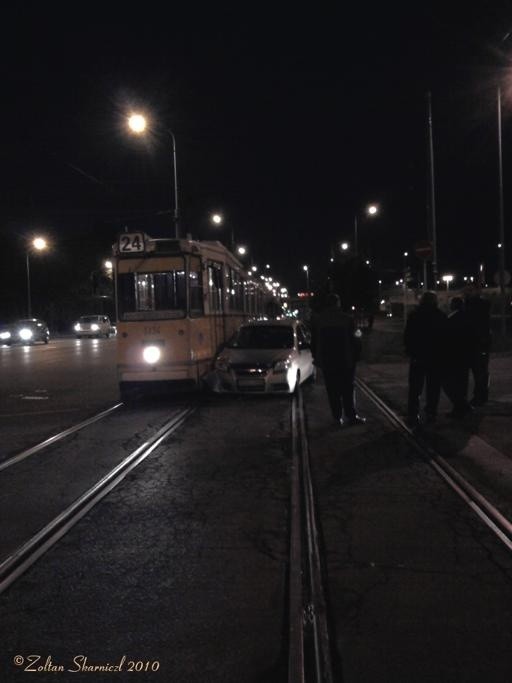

[0,319,49,346]
[74,315,116,338]
[213,320,317,394]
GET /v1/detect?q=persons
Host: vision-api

[310,289,367,425]
[403,285,492,424]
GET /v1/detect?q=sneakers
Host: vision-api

[328,398,489,428]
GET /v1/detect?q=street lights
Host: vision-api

[27,239,46,319]
[212,205,502,298]
[128,114,179,238]
[91,261,112,296]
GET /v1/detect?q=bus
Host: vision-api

[112,230,274,398]
